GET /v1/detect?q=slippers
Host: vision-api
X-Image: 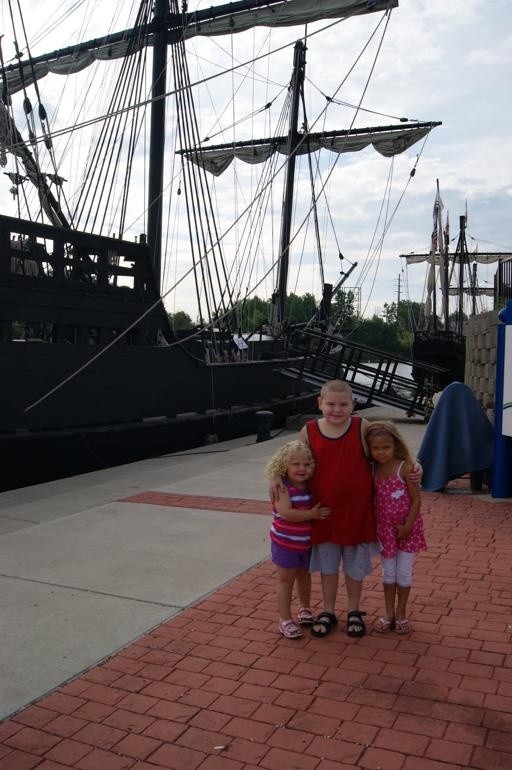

[375,618,392,632]
[395,619,410,634]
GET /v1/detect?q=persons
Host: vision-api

[269,379,423,638]
[364,420,428,634]
[262,440,330,639]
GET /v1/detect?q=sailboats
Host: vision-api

[0,1,443,490]
[398,178,512,393]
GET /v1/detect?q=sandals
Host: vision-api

[311,612,337,637]
[348,611,366,637]
[278,619,303,638]
[297,606,313,624]
